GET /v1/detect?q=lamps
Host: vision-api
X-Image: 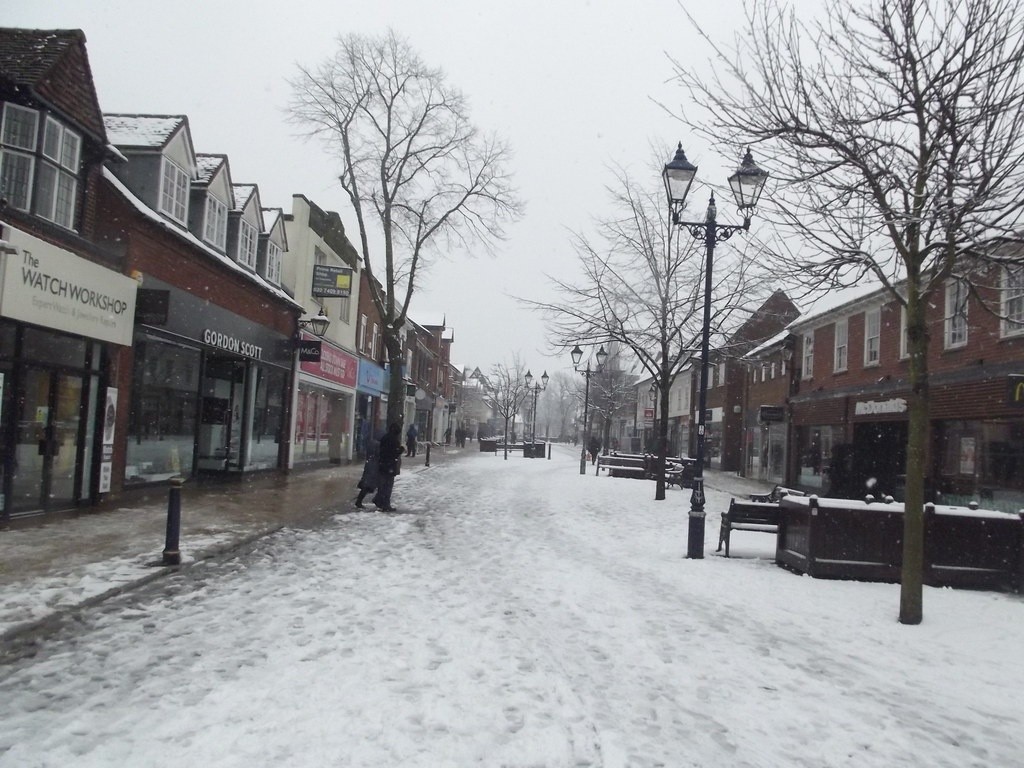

[879,374,891,385]
[300,306,330,336]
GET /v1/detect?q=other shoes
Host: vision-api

[383,505,396,511]
[355,502,367,509]
[372,497,382,508]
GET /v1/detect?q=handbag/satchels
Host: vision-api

[586,452,591,461]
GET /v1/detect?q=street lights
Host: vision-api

[570,341,608,477]
[524,370,549,459]
[661,140,769,561]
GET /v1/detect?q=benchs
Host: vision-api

[666,463,685,490]
[596,455,648,480]
[717,497,779,558]
[750,484,810,502]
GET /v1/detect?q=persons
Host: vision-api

[443,427,475,449]
[587,434,602,466]
[377,423,406,512]
[405,421,417,457]
[353,442,383,510]
